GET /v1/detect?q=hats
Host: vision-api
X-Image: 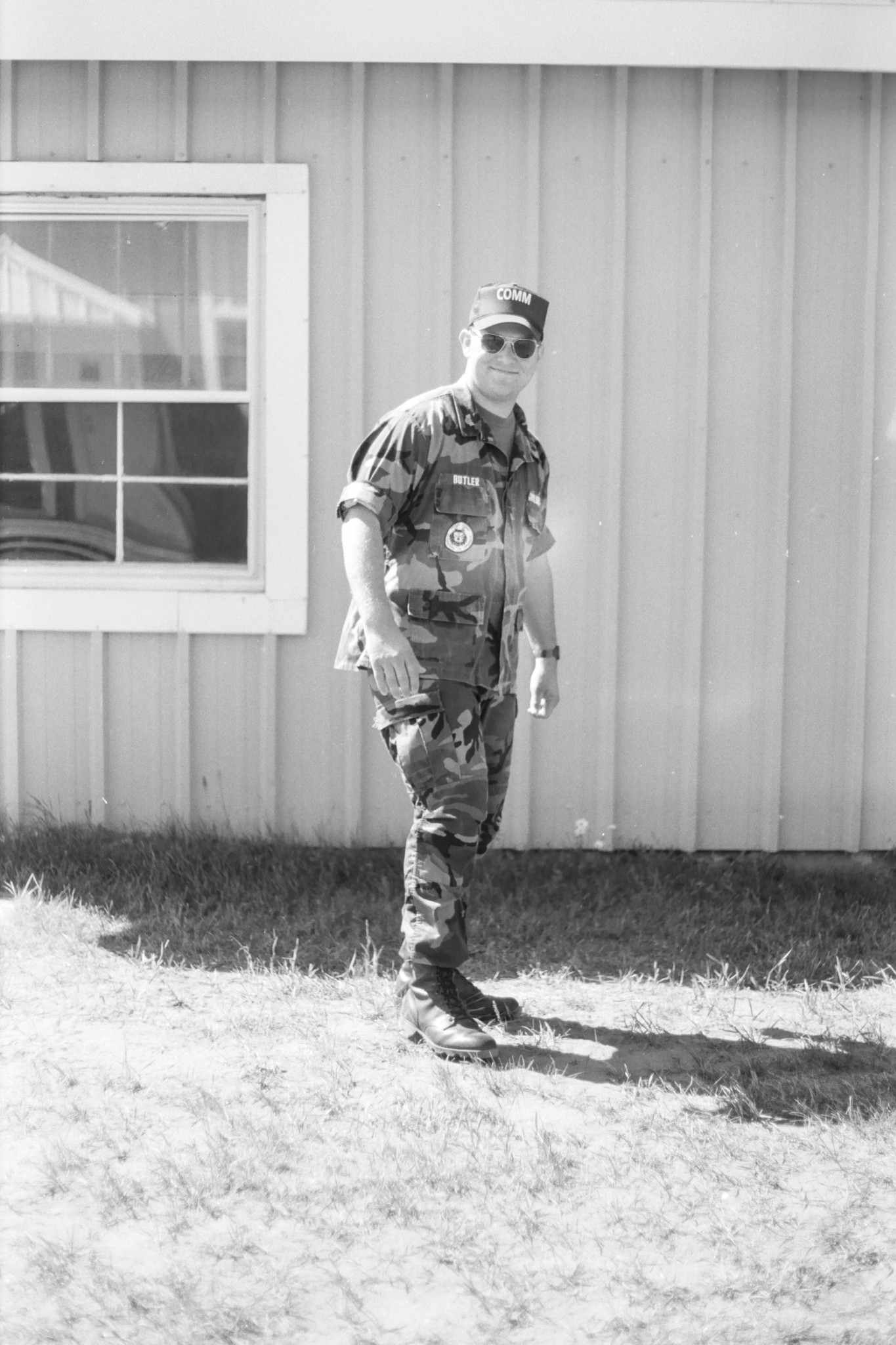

[471,282,549,342]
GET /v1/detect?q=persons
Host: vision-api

[337,282,560,1059]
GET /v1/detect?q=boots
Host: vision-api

[399,963,497,1059]
[394,961,519,1019]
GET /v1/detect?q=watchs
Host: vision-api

[534,646,560,660]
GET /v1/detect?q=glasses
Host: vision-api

[470,327,539,359]
